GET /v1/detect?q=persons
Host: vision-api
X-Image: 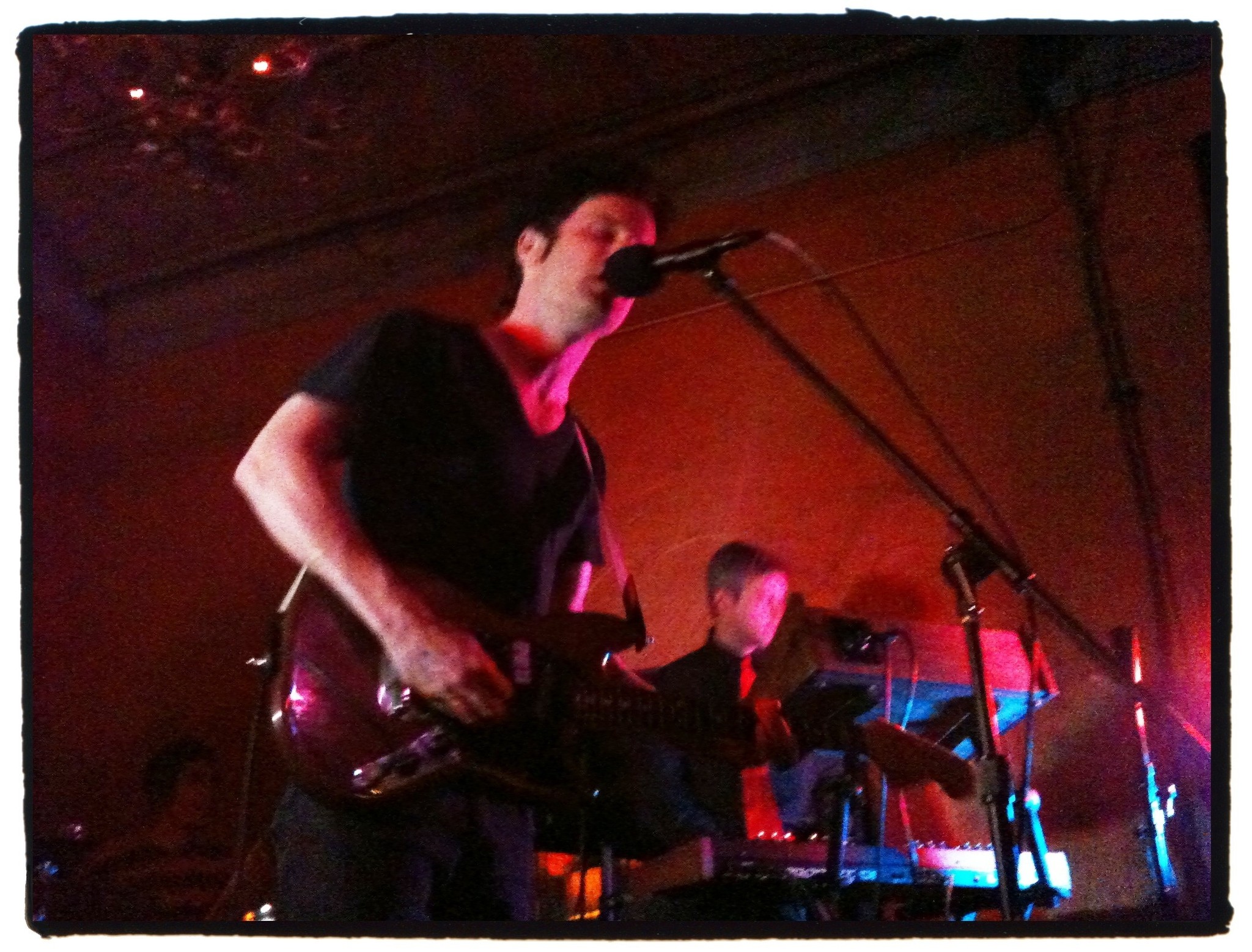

[80,740,264,916]
[230,174,657,921]
[612,543,816,856]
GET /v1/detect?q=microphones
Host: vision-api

[822,614,884,662]
[602,225,768,298]
[940,543,1002,600]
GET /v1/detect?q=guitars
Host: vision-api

[263,555,978,805]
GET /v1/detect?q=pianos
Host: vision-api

[624,833,1074,940]
